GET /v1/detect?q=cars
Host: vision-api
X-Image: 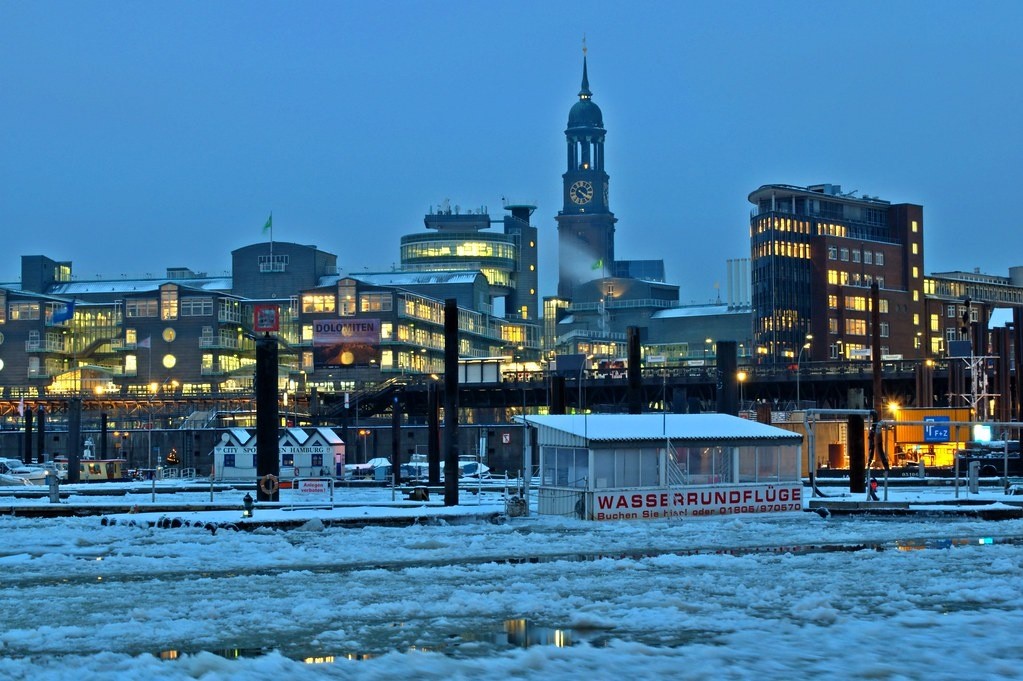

[418,459,494,485]
[0,457,63,487]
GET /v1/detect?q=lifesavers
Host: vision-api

[294,468,299,476]
[260,474,278,495]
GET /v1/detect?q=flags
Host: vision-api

[262,215,271,236]
[591,257,603,270]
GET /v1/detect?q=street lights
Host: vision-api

[113,431,129,459]
[360,429,371,463]
[926,359,935,407]
[737,369,749,412]
[577,354,594,413]
[795,342,811,411]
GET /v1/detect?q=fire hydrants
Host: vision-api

[243,492,255,517]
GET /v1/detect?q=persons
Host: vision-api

[895,442,917,466]
[870,476,877,492]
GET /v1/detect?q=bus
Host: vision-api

[53,457,128,484]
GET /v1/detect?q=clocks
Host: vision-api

[569,180,593,206]
